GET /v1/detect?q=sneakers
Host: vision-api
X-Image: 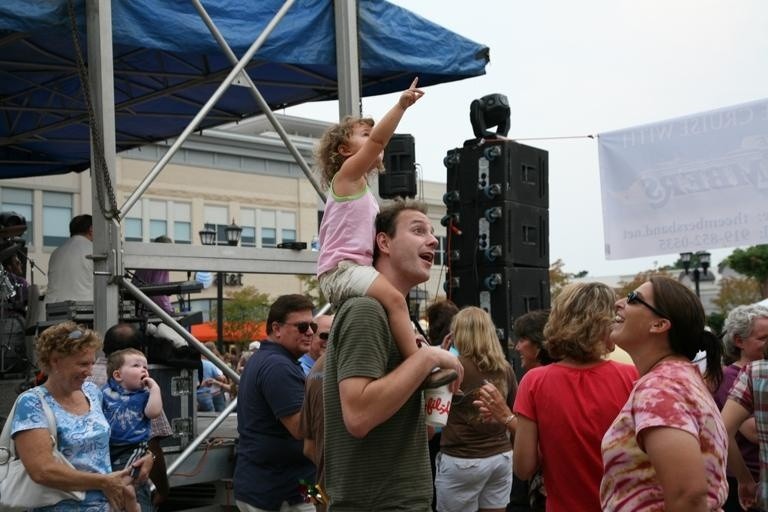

[419,367,458,389]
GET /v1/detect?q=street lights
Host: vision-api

[196,216,245,355]
[678,248,714,299]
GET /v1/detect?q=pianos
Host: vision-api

[120,279,202,299]
[123,310,203,327]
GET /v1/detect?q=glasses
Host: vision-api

[59,330,82,348]
[317,332,329,340]
[281,321,318,334]
[626,290,665,318]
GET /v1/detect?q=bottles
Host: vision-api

[0,264,17,299]
[311,233,321,252]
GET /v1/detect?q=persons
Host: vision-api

[317,75,457,388]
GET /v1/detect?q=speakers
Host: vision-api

[378,135,417,197]
[441,208,548,267]
[442,268,549,351]
[442,138,549,208]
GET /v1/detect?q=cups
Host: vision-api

[424,382,454,427]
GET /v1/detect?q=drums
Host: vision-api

[1,312,26,374]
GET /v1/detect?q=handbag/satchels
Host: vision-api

[0,386,85,511]
